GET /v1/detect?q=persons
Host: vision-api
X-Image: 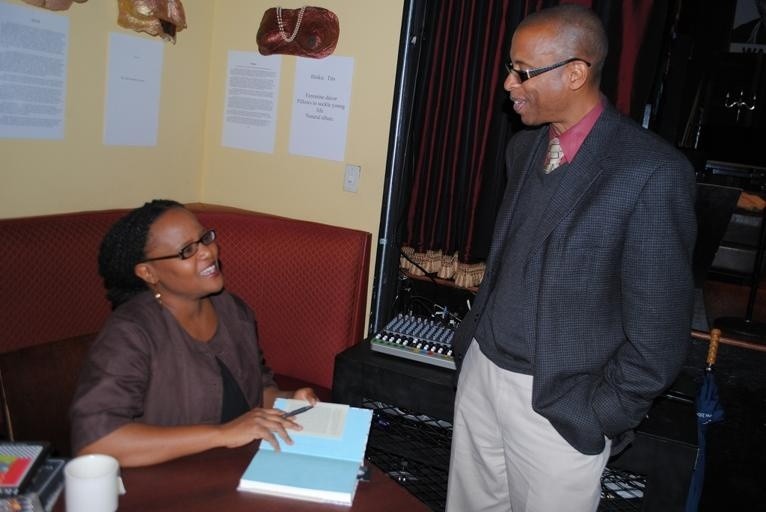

[444,3,698,512]
[67,197,321,468]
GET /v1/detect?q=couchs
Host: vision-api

[0,201,373,438]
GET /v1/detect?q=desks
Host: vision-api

[51,424,433,511]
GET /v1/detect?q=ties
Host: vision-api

[543,137,565,176]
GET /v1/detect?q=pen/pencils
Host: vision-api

[281,406,314,418]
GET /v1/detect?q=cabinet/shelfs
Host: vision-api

[328,324,707,511]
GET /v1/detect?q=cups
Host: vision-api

[62,453,120,512]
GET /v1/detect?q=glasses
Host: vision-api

[138,229,216,264]
[503,57,591,84]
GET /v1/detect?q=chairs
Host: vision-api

[0,332,108,441]
[695,158,766,327]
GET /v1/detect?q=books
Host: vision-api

[235,395,374,507]
[0,454,63,512]
[0,441,47,492]
